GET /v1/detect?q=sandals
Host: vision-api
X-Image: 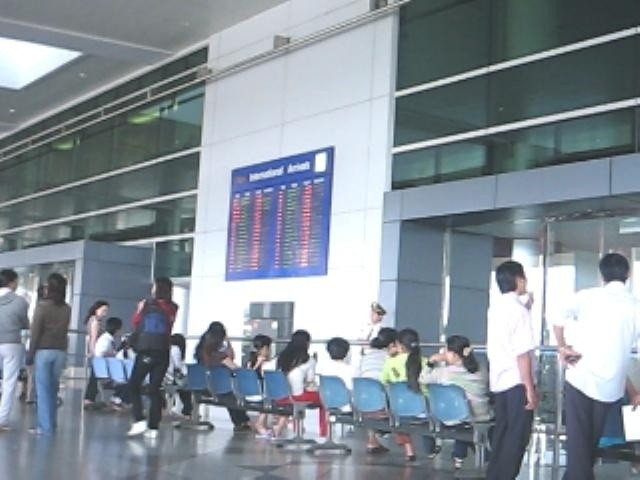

[427,445,441,459]
[453,456,463,469]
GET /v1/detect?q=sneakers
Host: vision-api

[404,455,416,463]
[143,429,159,438]
[127,420,147,436]
[367,445,391,453]
[233,425,252,433]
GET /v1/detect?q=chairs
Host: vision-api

[303,373,355,457]
[424,379,495,471]
[386,379,432,441]
[349,375,391,437]
[93,355,163,425]
[232,366,261,414]
[173,361,214,431]
[261,368,318,451]
[205,363,234,414]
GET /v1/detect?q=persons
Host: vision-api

[0,269,76,438]
[193,320,330,437]
[319,338,354,415]
[553,252,638,479]
[486,260,538,480]
[127,279,178,438]
[84,301,192,417]
[352,327,497,468]
[362,301,386,368]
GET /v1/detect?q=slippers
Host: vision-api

[255,429,272,438]
[271,432,290,441]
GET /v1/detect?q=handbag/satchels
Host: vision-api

[128,300,170,353]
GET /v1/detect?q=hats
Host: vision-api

[372,302,386,315]
